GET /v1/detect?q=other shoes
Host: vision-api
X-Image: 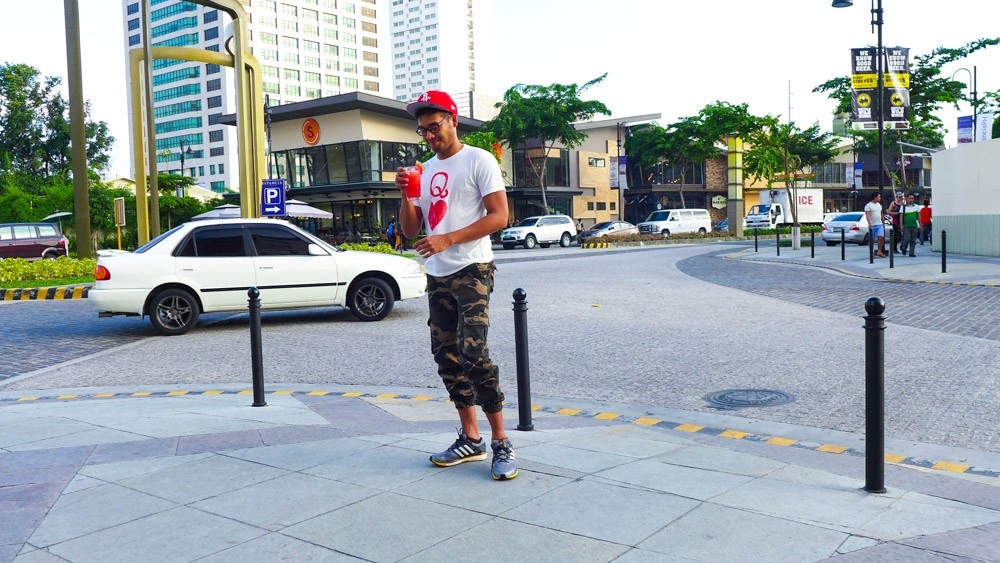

[909,254,916,257]
[900,247,908,252]
[894,249,899,254]
[902,250,906,255]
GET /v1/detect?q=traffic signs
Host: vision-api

[261,180,286,215]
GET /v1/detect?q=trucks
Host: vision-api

[744,188,824,230]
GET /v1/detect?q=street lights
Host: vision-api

[178,137,194,198]
[830,0,886,216]
[263,92,274,179]
[615,121,633,221]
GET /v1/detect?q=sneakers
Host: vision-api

[430,427,488,467]
[490,437,518,480]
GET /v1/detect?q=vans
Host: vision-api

[636,209,712,237]
[0,222,68,264]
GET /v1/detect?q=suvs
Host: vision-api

[499,214,577,250]
[87,217,429,337]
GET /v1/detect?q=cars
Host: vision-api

[712,218,730,233]
[576,221,640,241]
[822,211,902,246]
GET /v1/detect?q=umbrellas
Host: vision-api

[41,210,73,234]
[286,199,333,223]
[191,203,241,221]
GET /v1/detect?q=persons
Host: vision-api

[396,92,518,480]
[576,219,585,233]
[887,192,909,253]
[899,194,921,257]
[509,218,518,227]
[864,192,886,258]
[919,200,933,245]
[387,219,407,251]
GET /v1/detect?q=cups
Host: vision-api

[404,166,421,200]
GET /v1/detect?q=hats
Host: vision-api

[896,191,903,197]
[406,90,458,120]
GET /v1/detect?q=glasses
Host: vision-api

[416,115,448,136]
